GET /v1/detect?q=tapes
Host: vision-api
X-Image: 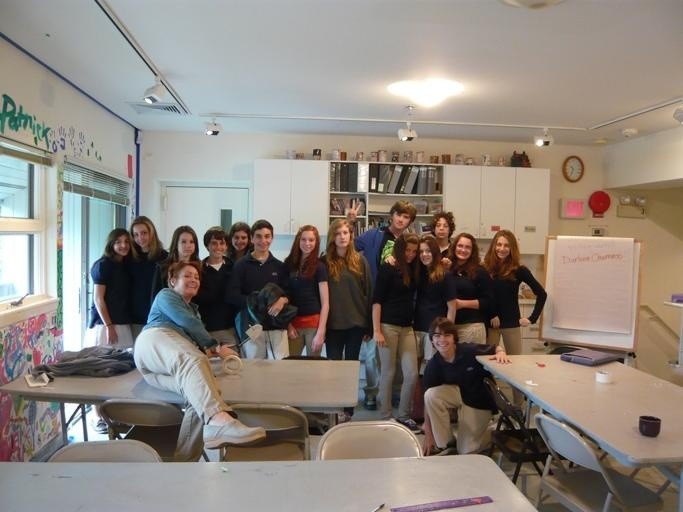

[596,371,610,383]
[222,355,242,375]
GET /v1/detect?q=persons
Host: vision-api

[349,201,547,434]
[134,260,265,463]
[91,216,289,361]
[422,317,513,457]
[282,219,373,416]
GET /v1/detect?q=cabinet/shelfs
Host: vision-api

[513,167,549,256]
[443,164,516,240]
[328,159,368,238]
[520,303,551,356]
[251,158,329,236]
[367,162,444,238]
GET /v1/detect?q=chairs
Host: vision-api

[217,401,312,460]
[280,354,340,437]
[317,421,422,461]
[99,398,210,464]
[42,440,165,465]
[480,374,569,492]
[535,411,663,512]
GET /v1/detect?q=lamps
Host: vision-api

[197,105,586,149]
[616,192,647,218]
[141,75,166,105]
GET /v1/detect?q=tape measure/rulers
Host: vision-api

[390,496,493,512]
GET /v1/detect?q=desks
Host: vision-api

[0,358,361,442]
[473,351,682,510]
[0,452,539,512]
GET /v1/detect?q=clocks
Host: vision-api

[562,155,583,184]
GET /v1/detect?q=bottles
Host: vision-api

[287,149,465,164]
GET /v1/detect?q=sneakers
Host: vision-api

[203,419,266,449]
[402,418,418,430]
[363,398,376,409]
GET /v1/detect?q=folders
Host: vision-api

[330,162,436,195]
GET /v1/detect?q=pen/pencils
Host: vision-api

[372,504,384,512]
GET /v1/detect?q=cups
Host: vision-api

[638,414,661,437]
[465,151,505,166]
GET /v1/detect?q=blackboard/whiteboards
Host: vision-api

[538,235,644,353]
